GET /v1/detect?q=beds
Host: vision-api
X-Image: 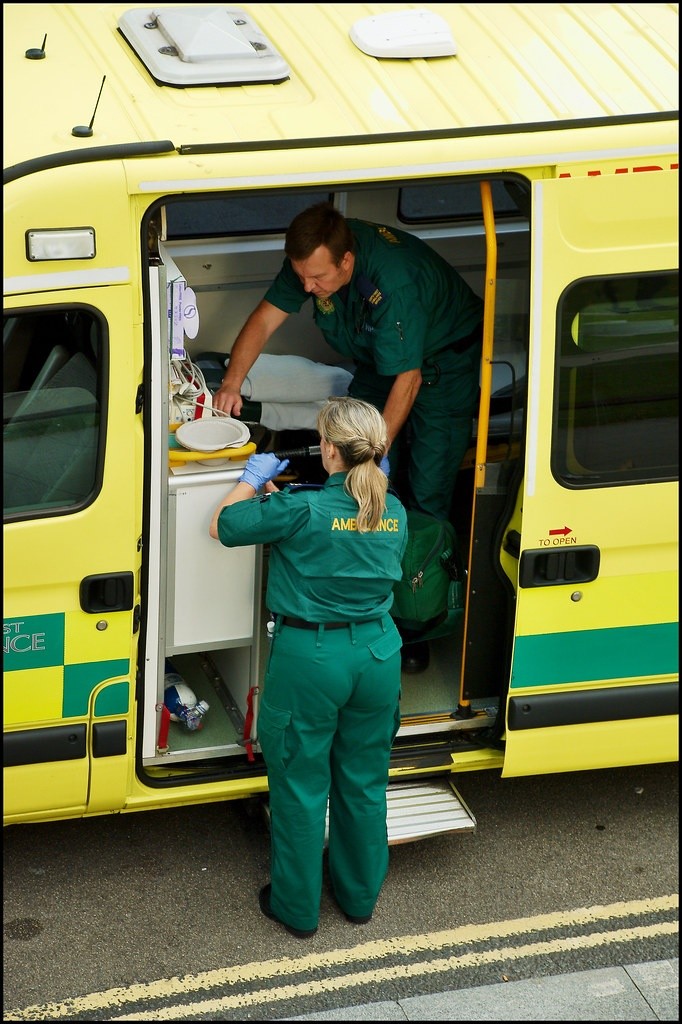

[193,339,531,484]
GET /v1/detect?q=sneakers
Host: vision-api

[322,847,372,923]
[259,882,318,938]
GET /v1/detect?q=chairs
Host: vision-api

[5,344,64,426]
[0,353,98,511]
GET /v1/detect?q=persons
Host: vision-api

[208,397,410,941]
[211,203,484,672]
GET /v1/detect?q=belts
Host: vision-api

[271,612,376,631]
[433,325,483,355]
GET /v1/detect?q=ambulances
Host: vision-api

[5,3,677,852]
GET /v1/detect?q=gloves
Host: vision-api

[237,453,289,492]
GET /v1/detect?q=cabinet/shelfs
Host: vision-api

[138,459,265,766]
